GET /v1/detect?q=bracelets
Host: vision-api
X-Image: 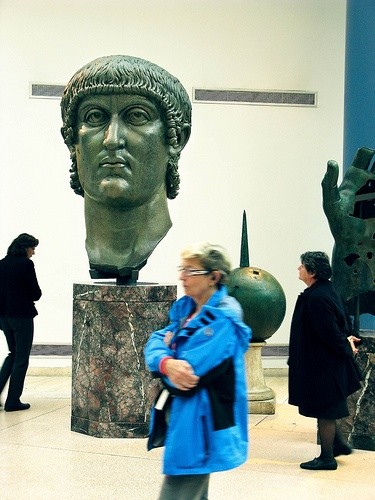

[159,357,172,377]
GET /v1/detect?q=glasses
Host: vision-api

[178,266,218,276]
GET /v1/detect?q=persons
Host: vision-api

[60,55,192,277]
[288,252,363,470]
[144,241,253,500]
[0,232,42,412]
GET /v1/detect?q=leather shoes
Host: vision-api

[6,403,30,411]
[335,445,353,457]
[300,457,338,471]
